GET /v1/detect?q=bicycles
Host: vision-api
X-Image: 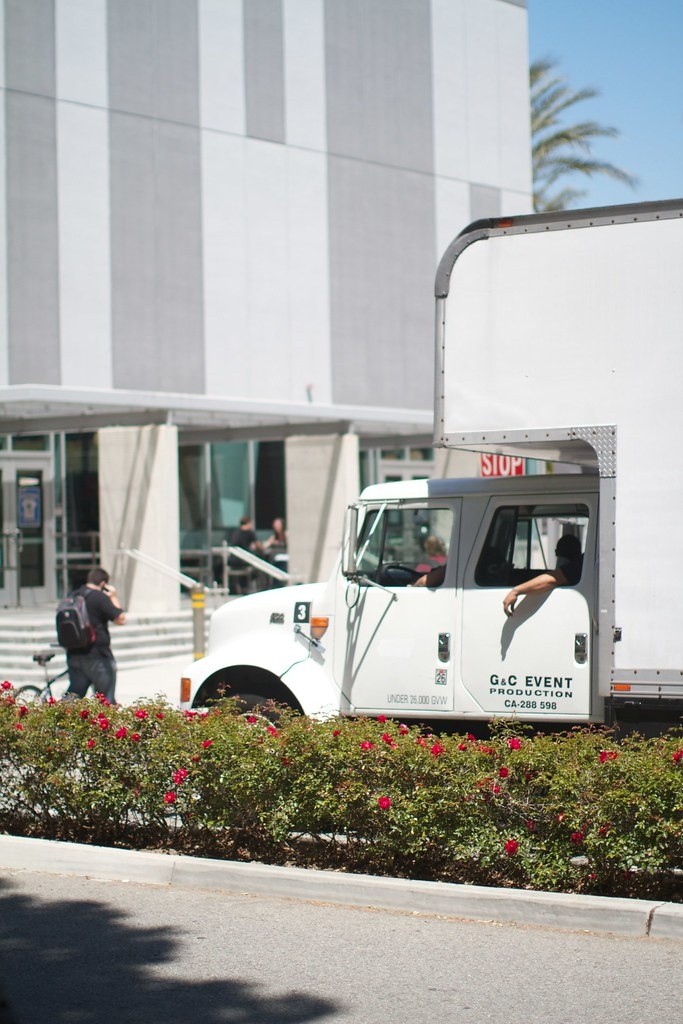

[12,642,66,711]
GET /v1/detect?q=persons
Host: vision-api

[415,535,451,587]
[58,568,125,708]
[504,535,586,616]
[225,517,288,594]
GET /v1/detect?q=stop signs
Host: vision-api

[475,452,529,481]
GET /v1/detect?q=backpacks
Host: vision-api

[56,588,96,651]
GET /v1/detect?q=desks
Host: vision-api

[266,548,289,589]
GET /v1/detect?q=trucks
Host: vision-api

[166,198,683,731]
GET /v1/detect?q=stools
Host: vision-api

[227,568,253,595]
